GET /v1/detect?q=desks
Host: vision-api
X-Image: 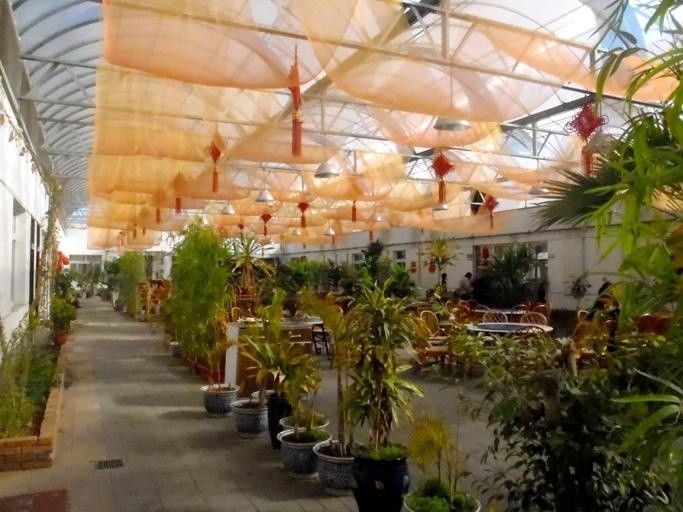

[461,323,555,387]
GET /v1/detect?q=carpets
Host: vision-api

[0,487,70,512]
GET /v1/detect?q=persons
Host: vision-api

[586,280,619,352]
[436,273,448,293]
[457,272,472,299]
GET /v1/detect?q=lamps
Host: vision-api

[528,159,543,196]
[219,165,275,214]
[315,133,340,178]
[432,204,448,210]
[433,65,471,131]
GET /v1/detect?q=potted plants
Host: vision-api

[298,289,367,497]
[395,400,480,512]
[229,288,331,448]
[164,213,479,387]
[164,214,240,418]
[275,357,331,479]
[337,280,431,511]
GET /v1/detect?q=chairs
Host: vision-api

[458,299,673,337]
